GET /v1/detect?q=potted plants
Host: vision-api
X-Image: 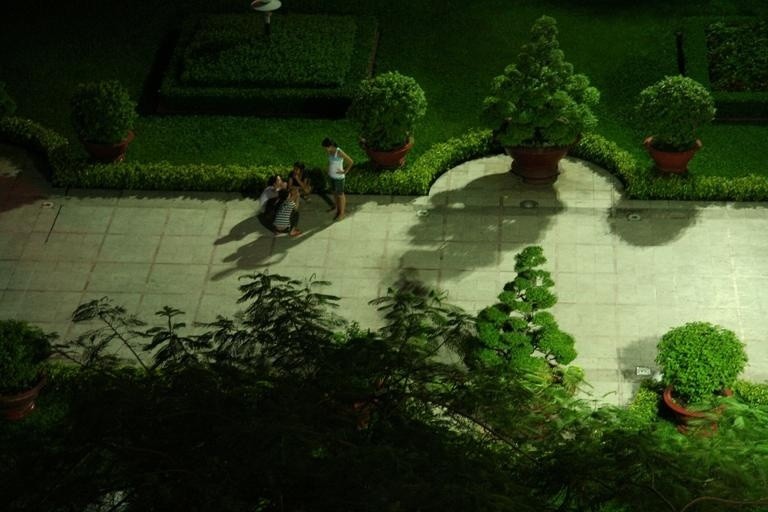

[477,17,601,179]
[0,320,54,421]
[60,79,139,168]
[347,72,428,169]
[629,74,717,172]
[654,319,748,436]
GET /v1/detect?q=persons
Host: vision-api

[256,175,301,237]
[287,162,314,204]
[321,137,354,222]
